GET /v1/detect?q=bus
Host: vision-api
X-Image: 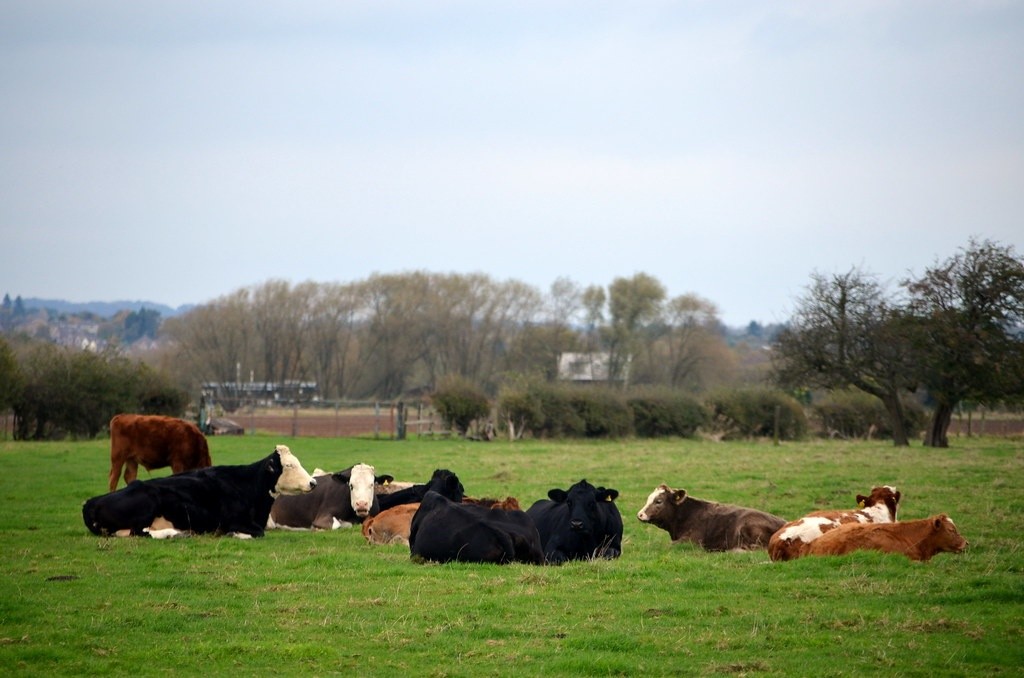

[201,382,320,411]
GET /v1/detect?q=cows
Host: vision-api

[83,413,967,567]
